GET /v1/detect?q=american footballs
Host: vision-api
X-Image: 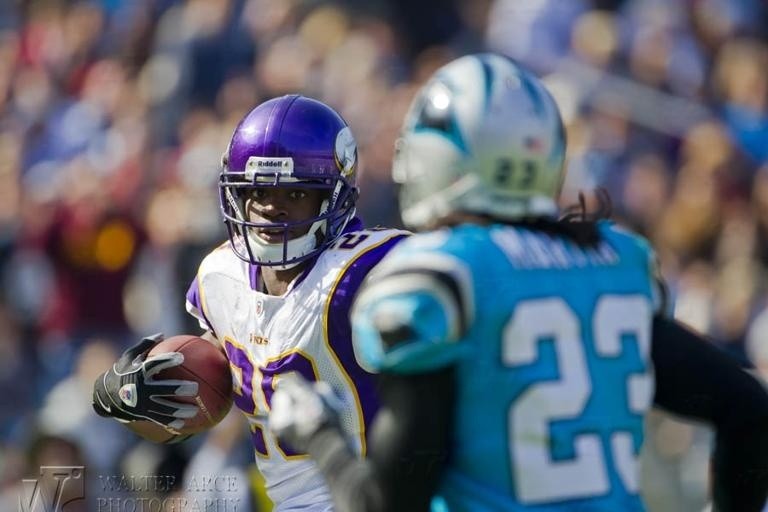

[146,335,232,430]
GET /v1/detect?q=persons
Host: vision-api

[93,52,768,512]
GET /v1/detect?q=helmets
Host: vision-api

[397,50,568,225]
[222,92,361,238]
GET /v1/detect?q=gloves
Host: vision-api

[265,370,344,450]
[89,329,201,434]
